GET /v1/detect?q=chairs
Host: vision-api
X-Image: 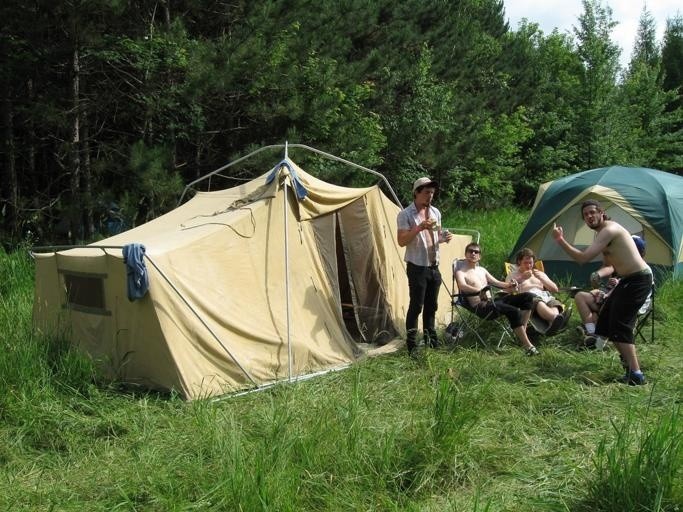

[573,282,655,353]
[502,260,569,344]
[450,257,518,350]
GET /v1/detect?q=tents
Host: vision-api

[502,161,682,286]
[25,143,455,407]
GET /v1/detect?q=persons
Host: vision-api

[396,177,454,359]
[456,239,540,358]
[573,236,654,351]
[505,248,574,336]
[546,199,654,386]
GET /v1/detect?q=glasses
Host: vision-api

[468,249,480,253]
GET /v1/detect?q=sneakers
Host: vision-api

[613,371,647,385]
[575,323,598,349]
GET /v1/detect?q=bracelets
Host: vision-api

[516,276,525,284]
[414,226,424,232]
[554,235,563,241]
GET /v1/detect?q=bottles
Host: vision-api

[510,279,520,296]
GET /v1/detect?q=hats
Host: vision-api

[413,177,439,192]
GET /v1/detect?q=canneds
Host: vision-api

[442,229,448,240]
